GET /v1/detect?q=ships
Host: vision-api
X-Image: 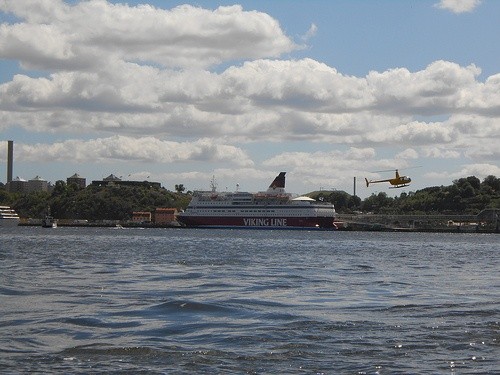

[174,171,339,231]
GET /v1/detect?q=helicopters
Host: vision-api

[365,166,424,190]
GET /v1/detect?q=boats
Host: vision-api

[114,225,124,230]
[41,216,58,228]
